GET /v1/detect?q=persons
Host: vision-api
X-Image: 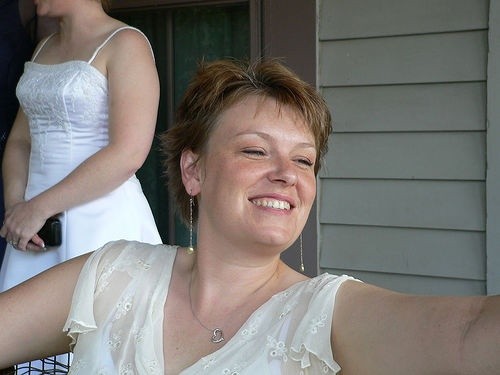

[0,57,500,374]
[0,0,164,292]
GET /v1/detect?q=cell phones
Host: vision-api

[28,217,62,246]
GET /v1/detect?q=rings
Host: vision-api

[12,240,17,245]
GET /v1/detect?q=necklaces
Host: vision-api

[187,259,224,343]
